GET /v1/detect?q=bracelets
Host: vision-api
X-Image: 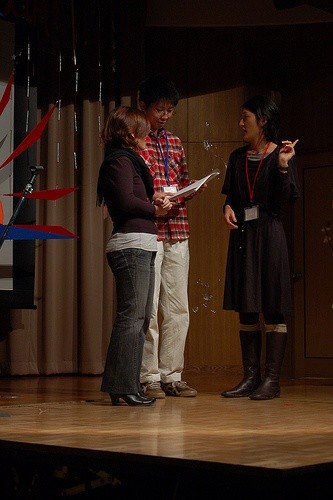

[278,166,290,171]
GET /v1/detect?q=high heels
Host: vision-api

[109,391,156,406]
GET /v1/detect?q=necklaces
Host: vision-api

[254,150,260,155]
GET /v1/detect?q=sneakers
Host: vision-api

[161,381,197,396]
[141,382,165,398]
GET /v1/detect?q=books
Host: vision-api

[167,171,220,201]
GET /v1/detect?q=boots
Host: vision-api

[220,330,262,397]
[249,332,287,399]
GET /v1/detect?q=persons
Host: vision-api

[130,77,208,398]
[220,96,299,400]
[96,106,173,406]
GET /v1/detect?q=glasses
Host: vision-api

[150,104,177,114]
